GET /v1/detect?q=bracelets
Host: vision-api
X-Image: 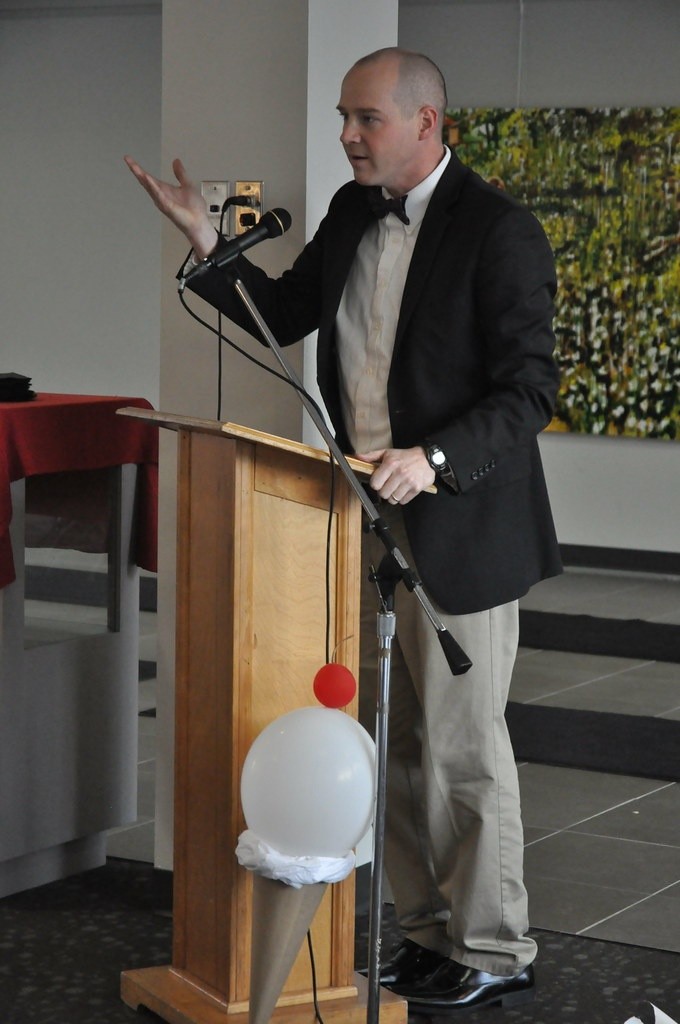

[191,251,199,266]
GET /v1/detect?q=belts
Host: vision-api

[361,479,385,506]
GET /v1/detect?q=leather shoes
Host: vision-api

[386,959,536,1016]
[357,939,449,987]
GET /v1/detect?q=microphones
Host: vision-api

[184,208,292,281]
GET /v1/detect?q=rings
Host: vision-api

[391,494,401,502]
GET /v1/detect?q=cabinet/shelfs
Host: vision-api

[0,390,144,898]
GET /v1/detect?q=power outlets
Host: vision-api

[230,180,265,237]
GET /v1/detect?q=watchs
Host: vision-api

[414,437,448,483]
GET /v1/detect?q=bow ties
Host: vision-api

[367,190,409,225]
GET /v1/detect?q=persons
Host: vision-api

[124,47,565,1015]
